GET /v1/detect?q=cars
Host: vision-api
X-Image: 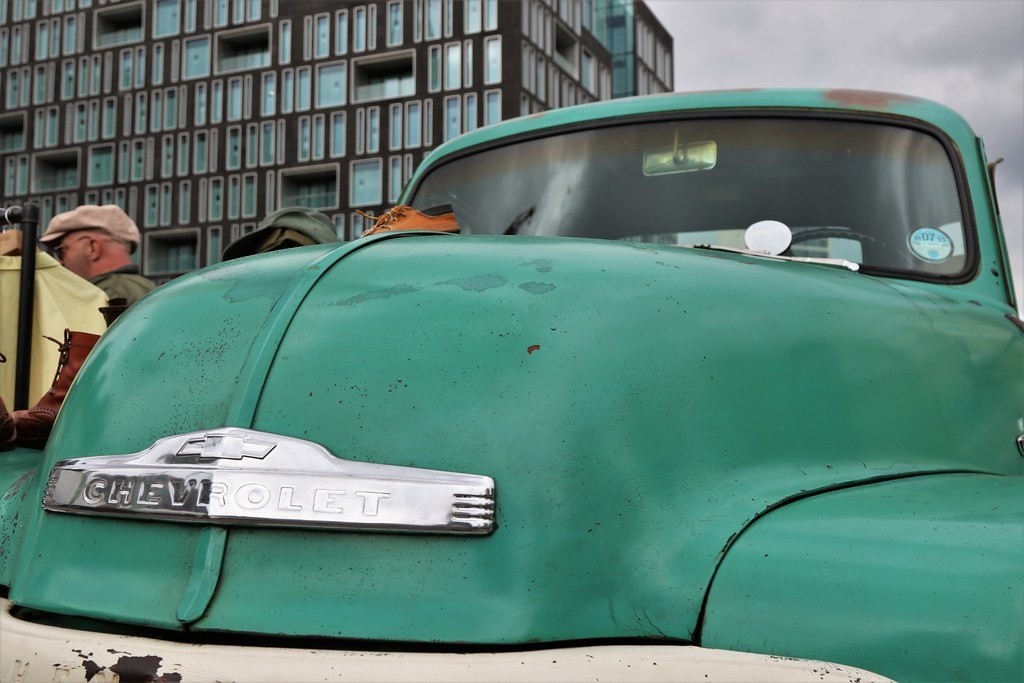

[1,79,1024,682]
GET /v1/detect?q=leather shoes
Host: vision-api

[356,204,461,238]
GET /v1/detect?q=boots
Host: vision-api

[10,328,101,451]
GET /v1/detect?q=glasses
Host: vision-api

[38,205,139,256]
[53,236,91,260]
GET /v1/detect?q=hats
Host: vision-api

[221,206,338,262]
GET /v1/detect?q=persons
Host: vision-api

[39,204,157,309]
